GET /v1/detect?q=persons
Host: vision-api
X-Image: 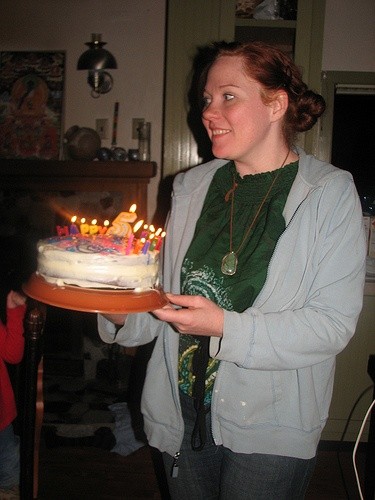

[0,290,28,500]
[94,36,367,500]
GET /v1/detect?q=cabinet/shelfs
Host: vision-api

[0,160,157,292]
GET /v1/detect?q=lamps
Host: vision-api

[76,31,118,98]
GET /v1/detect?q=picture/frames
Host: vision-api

[0,50,67,160]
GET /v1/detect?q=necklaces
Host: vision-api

[221,147,291,275]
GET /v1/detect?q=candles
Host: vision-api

[56,204,166,254]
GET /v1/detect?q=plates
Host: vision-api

[61,125,102,161]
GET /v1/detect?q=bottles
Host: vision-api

[128,148,140,161]
[113,151,126,161]
[137,121,151,162]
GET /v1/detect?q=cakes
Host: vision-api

[37,234,161,290]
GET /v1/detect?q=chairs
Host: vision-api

[18,303,51,499]
